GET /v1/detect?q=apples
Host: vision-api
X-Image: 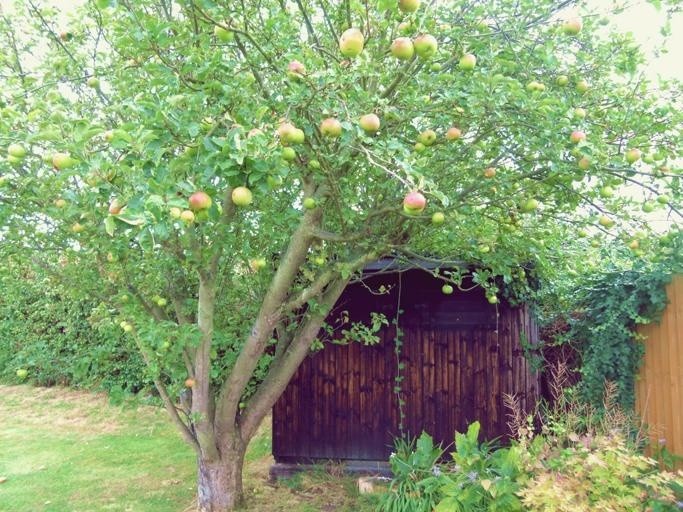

[0,0,683,388]
